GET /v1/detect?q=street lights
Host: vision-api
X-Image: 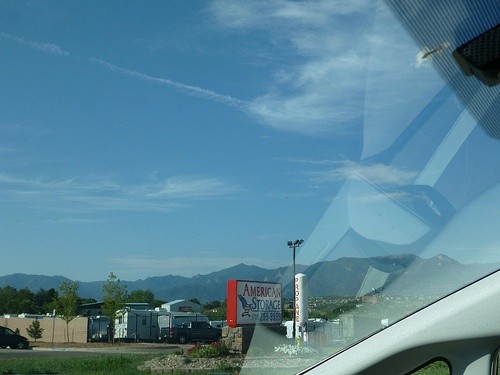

[287,239,304,344]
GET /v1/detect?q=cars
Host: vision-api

[0,325,27,348]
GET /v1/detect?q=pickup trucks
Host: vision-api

[160,320,223,344]
